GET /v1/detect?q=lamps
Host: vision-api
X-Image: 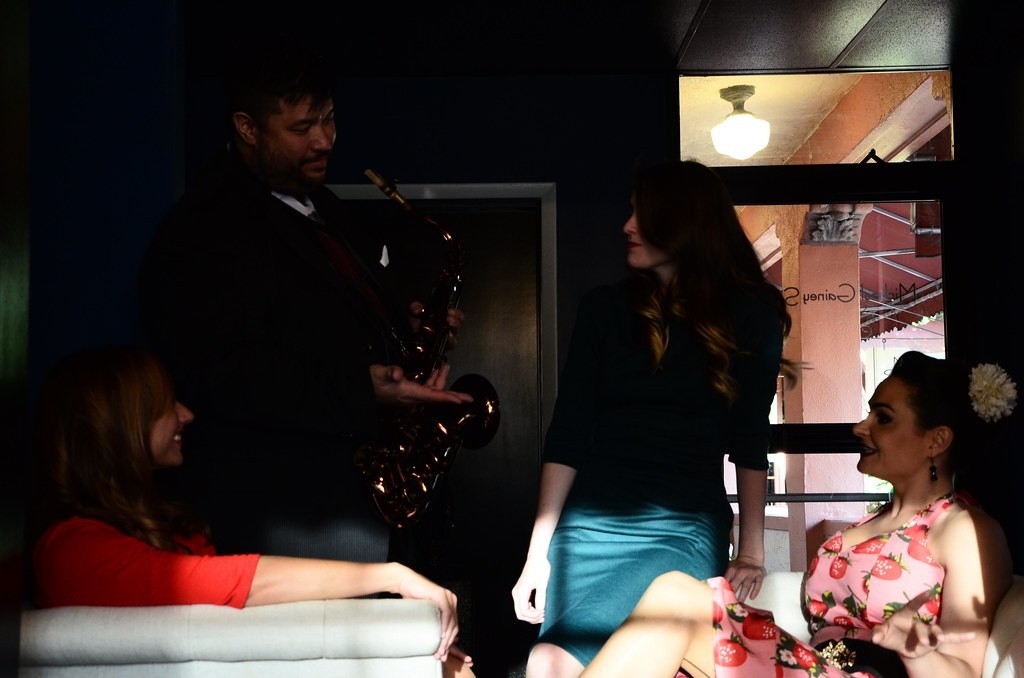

[711,84,771,162]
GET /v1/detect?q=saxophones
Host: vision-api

[364,162,502,531]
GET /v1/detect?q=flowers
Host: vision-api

[968,363,1017,424]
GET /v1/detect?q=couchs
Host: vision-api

[19,599,444,678]
[716,574,1024,678]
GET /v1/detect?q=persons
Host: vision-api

[136,55,474,560]
[31,345,477,678]
[577,350,1018,678]
[513,160,799,678]
